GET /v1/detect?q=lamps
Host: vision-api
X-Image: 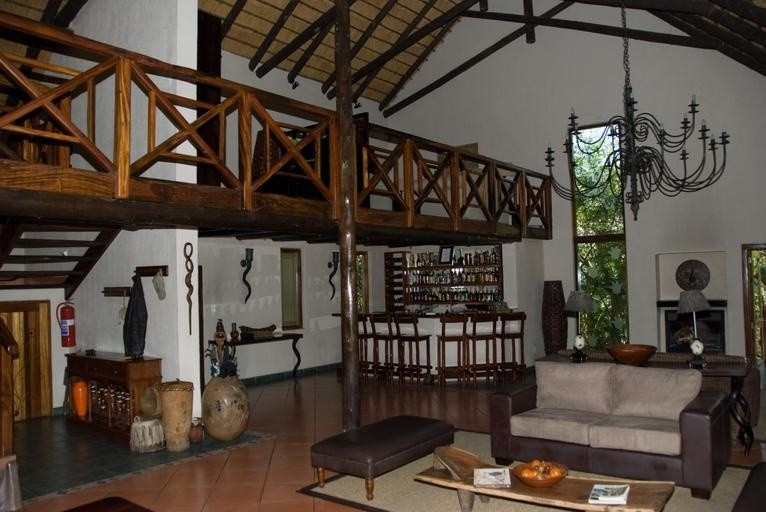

[545,8,730,222]
[678,289,711,339]
[564,291,594,363]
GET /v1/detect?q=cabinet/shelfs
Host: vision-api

[332,314,521,382]
[385,245,503,313]
[64,354,162,436]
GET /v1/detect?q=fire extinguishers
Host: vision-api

[56,297,76,347]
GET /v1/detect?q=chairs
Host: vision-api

[466,313,499,386]
[358,314,381,385]
[496,312,527,386]
[394,314,432,385]
[369,313,406,387]
[435,314,471,388]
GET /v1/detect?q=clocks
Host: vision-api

[687,335,707,368]
[675,260,710,291]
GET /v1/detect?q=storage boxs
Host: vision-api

[655,251,728,307]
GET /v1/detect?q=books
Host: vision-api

[474,467,511,488]
[589,484,629,506]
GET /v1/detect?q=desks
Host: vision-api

[208,333,303,384]
[535,353,754,454]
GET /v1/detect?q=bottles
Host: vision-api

[406,266,503,284]
[408,248,501,266]
[404,288,510,303]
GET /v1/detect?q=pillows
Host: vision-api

[467,408,490,427]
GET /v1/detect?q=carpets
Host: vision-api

[13,414,280,505]
[295,428,757,512]
[60,496,154,512]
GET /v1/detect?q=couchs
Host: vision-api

[489,361,732,501]
[310,413,454,501]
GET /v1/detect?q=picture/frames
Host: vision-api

[659,307,728,354]
[438,245,454,264]
